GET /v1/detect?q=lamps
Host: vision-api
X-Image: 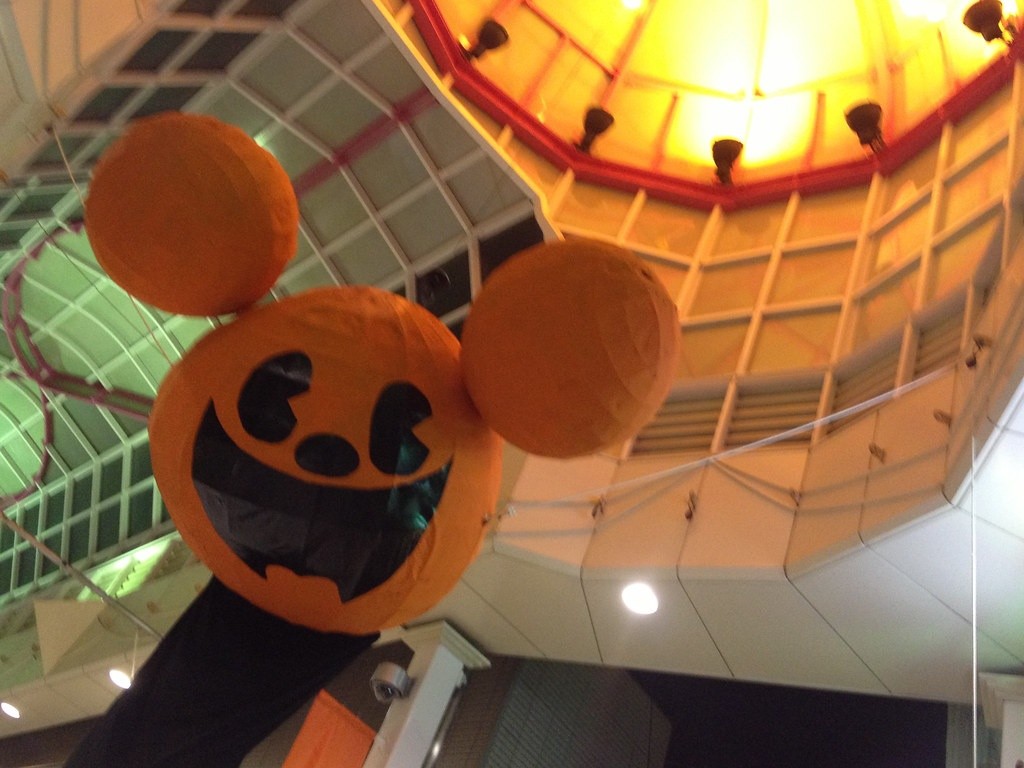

[461,20,511,66]
[960,1,1009,46]
[713,140,744,184]
[846,101,889,158]
[575,110,614,153]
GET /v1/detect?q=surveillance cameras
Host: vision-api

[368,661,408,705]
